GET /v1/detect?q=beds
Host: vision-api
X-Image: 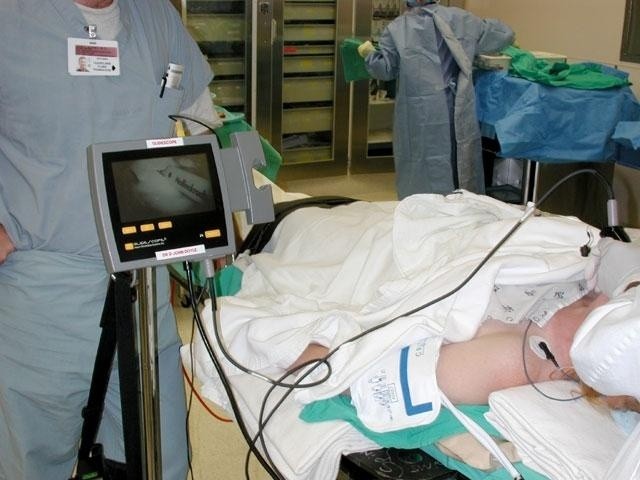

[190,219,640,478]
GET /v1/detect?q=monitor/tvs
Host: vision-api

[85,135,236,274]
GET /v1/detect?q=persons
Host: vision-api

[354,1,516,202]
[1,1,222,480]
[262,187,640,427]
[77,57,89,73]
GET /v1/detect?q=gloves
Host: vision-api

[358,40,374,57]
